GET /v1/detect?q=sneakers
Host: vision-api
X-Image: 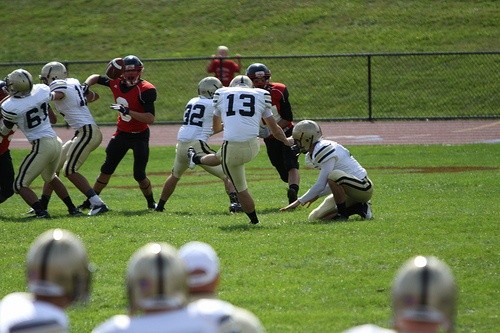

[78,201,91,209]
[28,211,49,219]
[27,208,47,214]
[229,202,242,212]
[334,214,349,221]
[356,201,373,220]
[188,145,196,169]
[147,201,157,209]
[68,208,82,216]
[87,204,109,216]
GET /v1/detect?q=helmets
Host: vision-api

[124,241,188,311]
[229,75,255,88]
[292,119,322,154]
[39,61,67,83]
[123,56,144,72]
[4,67,33,99]
[390,255,457,333]
[26,228,92,301]
[217,46,229,56]
[105,58,125,80]
[197,76,222,98]
[246,63,271,80]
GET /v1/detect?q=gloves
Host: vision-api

[81,83,89,96]
[110,103,129,116]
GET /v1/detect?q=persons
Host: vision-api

[246,62,301,204]
[78,54,157,211]
[0,80,15,203]
[153,76,244,213]
[91,242,239,333]
[26,61,109,216]
[0,68,77,219]
[207,45,241,87]
[187,74,295,224]
[179,241,265,333]
[342,255,456,333]
[0,228,91,333]
[279,120,374,222]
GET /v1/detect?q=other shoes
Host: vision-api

[288,188,297,209]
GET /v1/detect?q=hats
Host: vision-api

[179,240,219,288]
